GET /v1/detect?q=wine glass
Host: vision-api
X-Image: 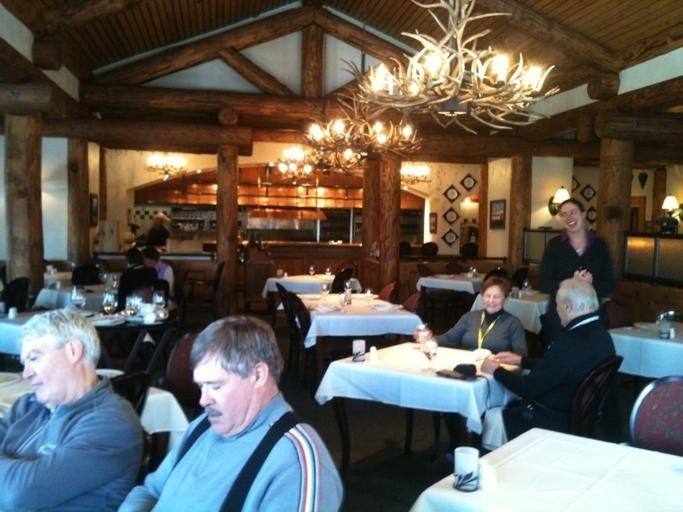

[69,270,165,316]
[422,339,438,373]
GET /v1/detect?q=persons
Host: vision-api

[0,308,144,512]
[480,277,616,458]
[414,275,528,455]
[118,248,158,311]
[538,198,616,351]
[141,247,175,298]
[147,213,170,246]
[117,314,344,512]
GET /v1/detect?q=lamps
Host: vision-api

[267,0,563,181]
[143,151,184,179]
[660,194,678,220]
[550,185,571,205]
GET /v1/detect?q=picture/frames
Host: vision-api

[488,199,506,230]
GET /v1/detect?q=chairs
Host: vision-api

[0,248,682,512]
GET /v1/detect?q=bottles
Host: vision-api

[369,344,378,366]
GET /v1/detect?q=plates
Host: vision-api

[312,303,339,314]
[371,304,402,312]
[0,371,20,387]
[434,274,455,279]
[633,322,652,330]
[87,317,125,327]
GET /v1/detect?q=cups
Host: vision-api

[454,446,479,490]
[7,307,17,320]
[276,266,373,306]
[46,266,53,275]
[657,319,671,338]
[468,265,530,298]
[416,325,428,352]
[352,340,365,362]
